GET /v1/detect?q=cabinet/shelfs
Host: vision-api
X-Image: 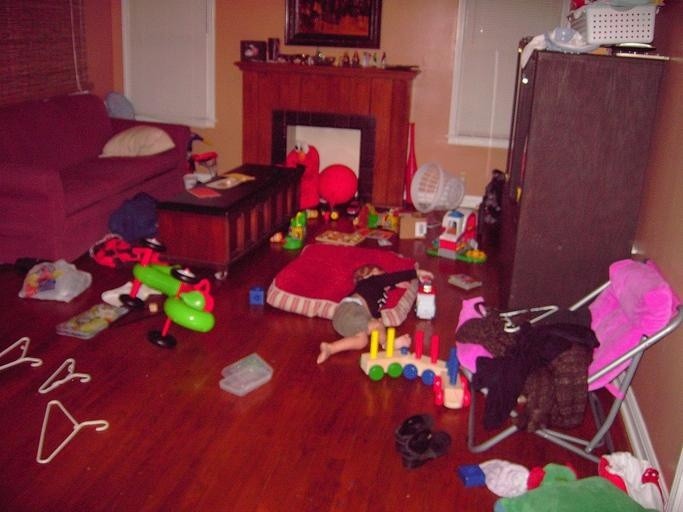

[500,41,670,309]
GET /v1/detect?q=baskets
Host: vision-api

[565,0,657,45]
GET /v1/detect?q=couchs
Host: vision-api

[0,94,190,265]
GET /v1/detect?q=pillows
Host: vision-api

[99,125,175,158]
[266,242,420,327]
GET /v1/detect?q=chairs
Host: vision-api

[468,254,682,463]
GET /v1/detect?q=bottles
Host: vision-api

[315,44,320,56]
[361,47,386,69]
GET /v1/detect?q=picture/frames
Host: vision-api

[285,0,382,49]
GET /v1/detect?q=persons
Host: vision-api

[315,264,422,365]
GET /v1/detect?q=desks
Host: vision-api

[155,162,301,280]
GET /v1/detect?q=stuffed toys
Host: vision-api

[284,142,358,209]
